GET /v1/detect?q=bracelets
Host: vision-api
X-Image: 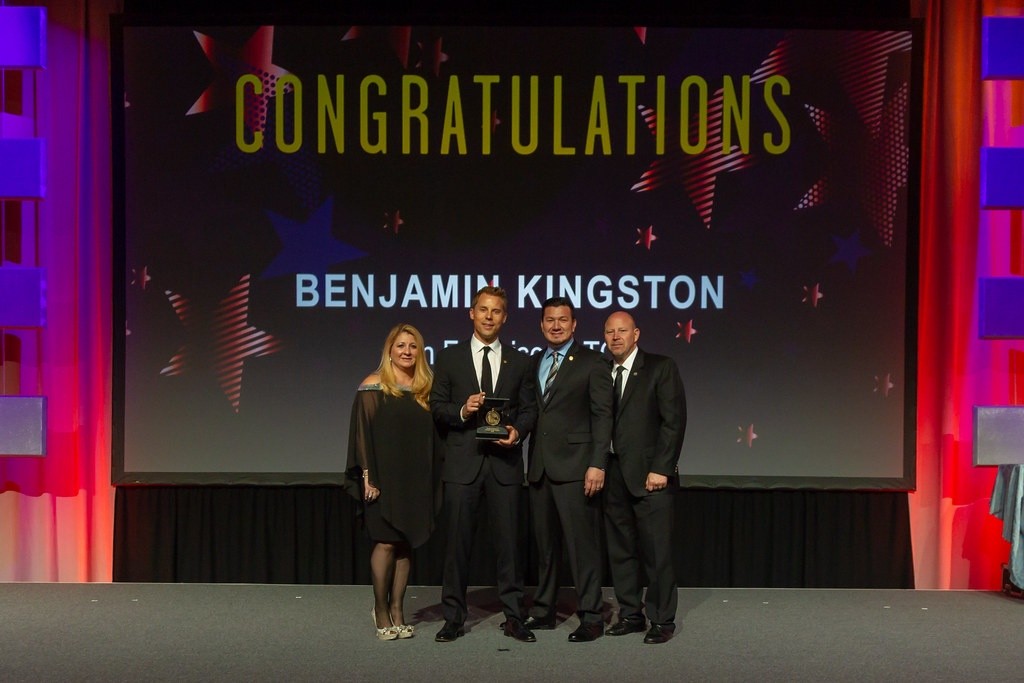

[362,470,368,477]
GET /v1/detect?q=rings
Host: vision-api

[368,496,371,497]
[596,489,600,490]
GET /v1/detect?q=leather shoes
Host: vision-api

[499,616,557,631]
[605,618,648,636]
[568,623,604,642]
[435,620,465,642]
[643,624,674,644]
[504,617,536,642]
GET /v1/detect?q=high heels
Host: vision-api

[371,605,414,641]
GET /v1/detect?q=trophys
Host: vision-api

[475,396,511,441]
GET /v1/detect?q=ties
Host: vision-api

[481,346,493,398]
[614,366,626,414]
[543,351,559,403]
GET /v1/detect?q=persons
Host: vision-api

[341,323,444,641]
[529,299,615,642]
[429,284,538,644]
[605,311,687,645]
